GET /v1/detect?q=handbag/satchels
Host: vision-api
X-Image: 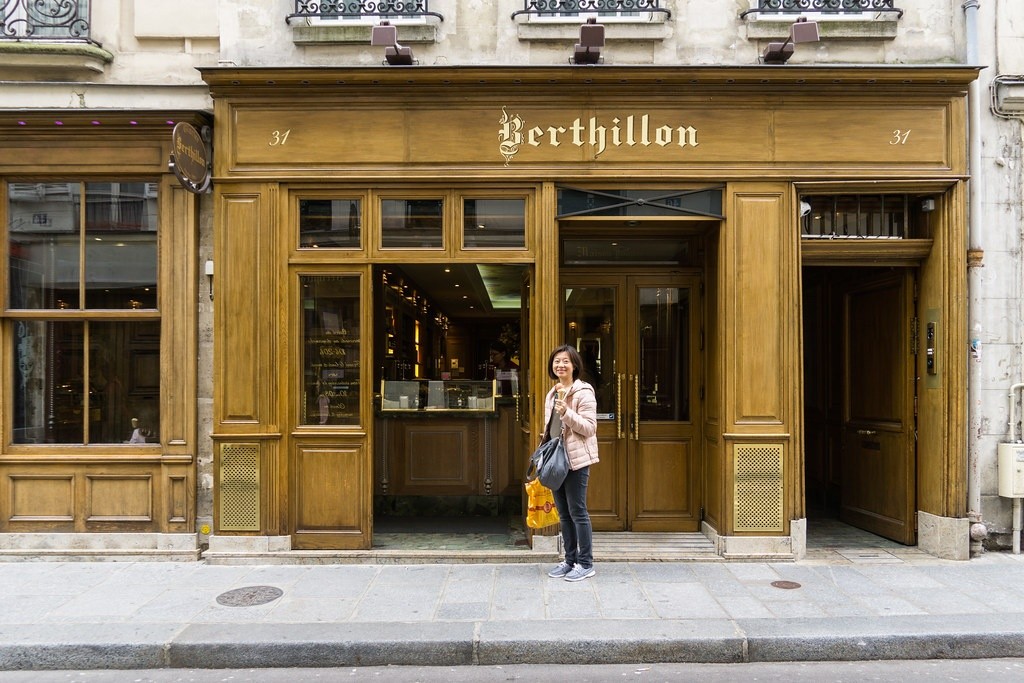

[525,477,560,528]
[526,433,571,491]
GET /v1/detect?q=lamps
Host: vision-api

[758,16,820,65]
[568,16,606,64]
[371,19,419,65]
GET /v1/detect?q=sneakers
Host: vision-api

[564,563,596,581]
[548,560,573,577]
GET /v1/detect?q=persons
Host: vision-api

[491,343,520,396]
[538,346,599,581]
[581,352,603,412]
